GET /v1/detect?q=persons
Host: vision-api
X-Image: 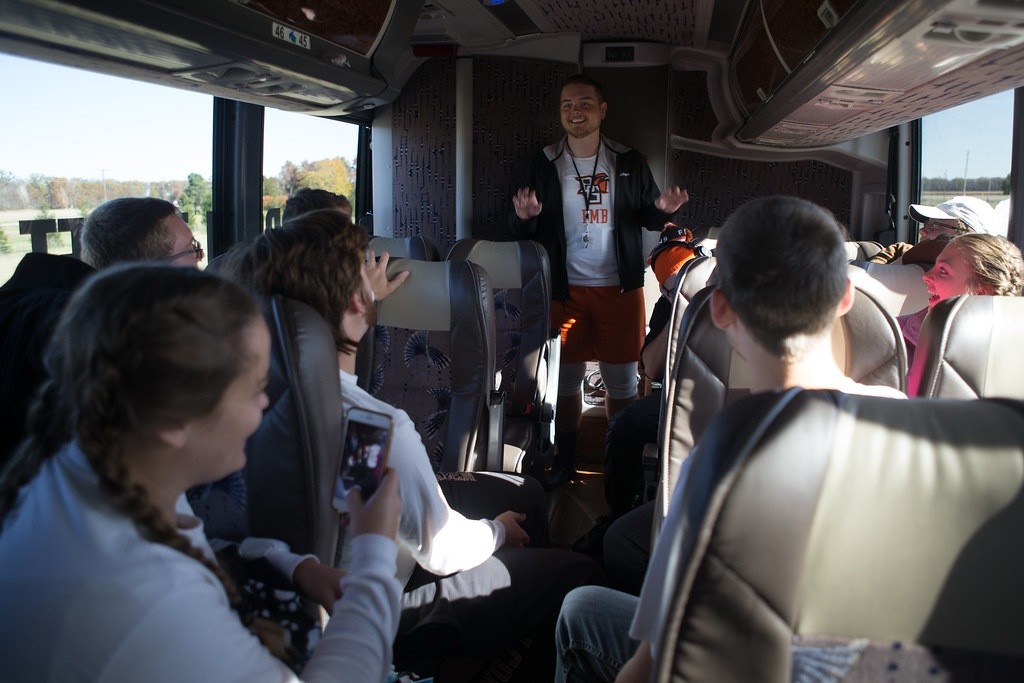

[79,198,203,271]
[508,75,689,492]
[0,264,404,683]
[282,190,351,225]
[894,233,1024,400]
[553,195,911,683]
[234,208,577,681]
[867,196,998,265]
[572,223,722,589]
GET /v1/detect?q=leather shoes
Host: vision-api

[572,518,617,554]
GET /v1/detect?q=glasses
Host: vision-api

[662,285,678,299]
[161,238,202,262]
[922,220,968,232]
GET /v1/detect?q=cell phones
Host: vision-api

[332,407,394,513]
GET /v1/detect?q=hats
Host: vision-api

[907,196,1000,234]
[651,241,698,286]
[659,222,687,241]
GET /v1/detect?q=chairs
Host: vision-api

[0,226,1024,683]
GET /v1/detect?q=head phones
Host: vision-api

[651,241,712,273]
